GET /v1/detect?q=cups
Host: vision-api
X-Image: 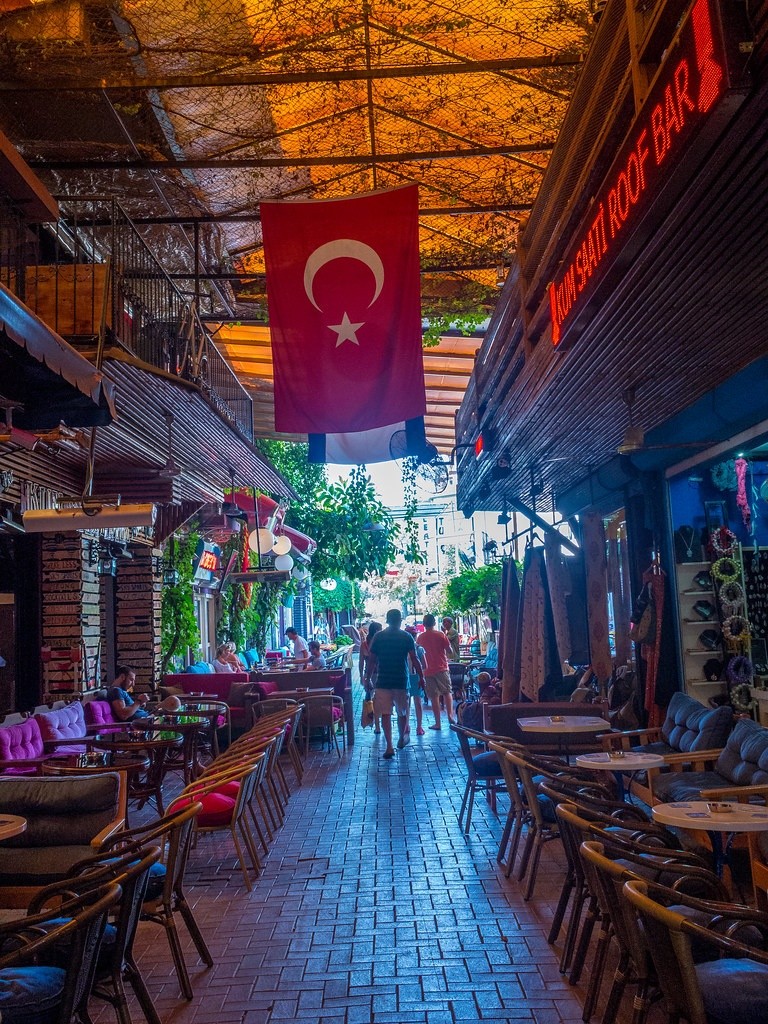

[250,661,257,671]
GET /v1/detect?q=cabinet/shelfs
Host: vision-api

[669,562,751,711]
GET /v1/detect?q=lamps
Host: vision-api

[23,503,158,530]
[85,538,117,578]
[231,570,292,584]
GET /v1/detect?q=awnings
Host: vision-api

[0,279,116,433]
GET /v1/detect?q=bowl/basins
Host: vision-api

[549,717,564,726]
[76,753,109,767]
[187,703,198,710]
[163,716,179,721]
[706,802,731,813]
[190,692,203,696]
[296,687,310,692]
[607,752,625,757]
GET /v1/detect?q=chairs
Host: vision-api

[446,687,768,1024]
[0,643,354,1024]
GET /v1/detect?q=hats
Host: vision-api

[405,627,420,633]
[283,627,296,635]
[386,609,402,624]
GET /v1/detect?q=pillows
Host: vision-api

[328,674,346,698]
[227,679,253,704]
[255,681,279,700]
[158,686,178,699]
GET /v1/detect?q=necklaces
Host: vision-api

[679,529,695,557]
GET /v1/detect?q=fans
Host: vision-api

[202,468,272,520]
[538,385,716,464]
[457,547,479,571]
[390,429,475,495]
[116,408,206,476]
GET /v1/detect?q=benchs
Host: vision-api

[473,700,609,815]
[156,671,255,733]
[0,771,130,909]
[258,669,347,749]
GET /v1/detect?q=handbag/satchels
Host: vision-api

[629,599,654,643]
[630,581,650,623]
[362,691,373,729]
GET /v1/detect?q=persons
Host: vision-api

[108,665,181,755]
[214,609,503,758]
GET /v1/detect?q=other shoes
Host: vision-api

[374,730,381,733]
[383,751,394,758]
[397,736,410,748]
[429,725,441,730]
[418,729,424,735]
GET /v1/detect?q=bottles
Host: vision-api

[563,661,570,674]
[626,657,632,672]
[151,714,154,722]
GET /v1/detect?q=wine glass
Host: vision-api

[41,646,51,695]
[70,644,82,695]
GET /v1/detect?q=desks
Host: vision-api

[92,731,184,810]
[130,716,210,777]
[158,703,226,758]
[0,814,26,841]
[576,751,664,801]
[513,715,609,767]
[39,753,151,789]
[266,688,334,751]
[650,801,768,879]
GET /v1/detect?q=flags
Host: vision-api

[259,178,428,434]
[306,415,429,466]
